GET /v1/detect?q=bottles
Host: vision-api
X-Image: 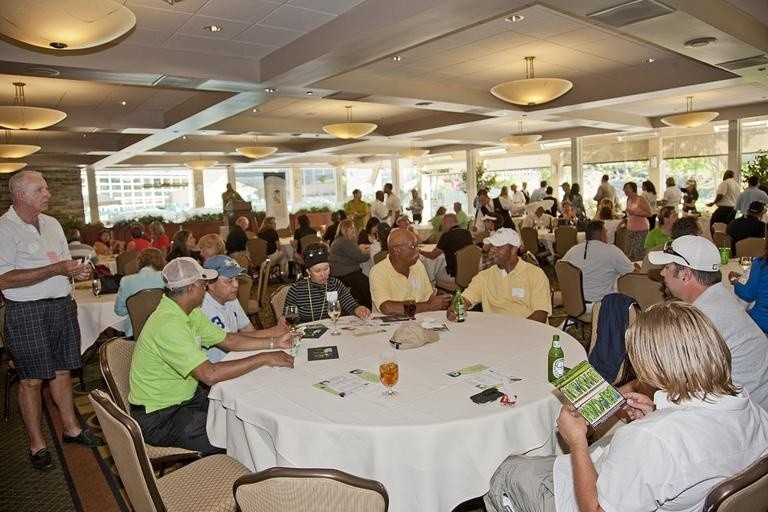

[548,335,564,382]
[548,217,554,233]
[453,288,465,322]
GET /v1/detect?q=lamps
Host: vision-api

[0,144,41,159]
[0,163,28,174]
[399,143,430,159]
[0,0,137,51]
[499,122,542,146]
[661,96,719,129]
[491,56,572,106]
[322,107,377,139]
[184,155,218,169]
[0,82,67,130]
[328,154,351,168]
[235,134,278,158]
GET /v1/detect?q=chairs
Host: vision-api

[89,389,253,512]
[233,467,390,512]
[555,213,768,339]
[116,243,270,340]
[0,305,19,424]
[98,337,198,463]
[271,227,555,323]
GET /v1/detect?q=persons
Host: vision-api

[127,256,294,455]
[0,168,106,473]
[419,169,768,415]
[67,167,453,364]
[486,300,767,511]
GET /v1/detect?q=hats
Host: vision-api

[203,254,247,278]
[161,256,219,291]
[482,227,522,247]
[390,322,440,350]
[648,234,722,272]
[749,200,766,214]
[480,214,498,221]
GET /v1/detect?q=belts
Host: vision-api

[129,404,145,412]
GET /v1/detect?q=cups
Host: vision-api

[540,221,546,232]
[719,247,731,265]
[403,295,417,321]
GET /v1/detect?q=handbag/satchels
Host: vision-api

[92,264,118,295]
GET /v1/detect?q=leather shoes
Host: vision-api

[29,447,56,472]
[62,429,104,446]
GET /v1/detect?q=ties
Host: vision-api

[487,202,493,211]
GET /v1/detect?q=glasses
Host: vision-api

[308,248,325,258]
[663,239,690,265]
[393,241,419,250]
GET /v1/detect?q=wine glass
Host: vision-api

[285,305,300,348]
[378,352,400,400]
[741,256,752,269]
[327,300,342,335]
[92,279,101,299]
